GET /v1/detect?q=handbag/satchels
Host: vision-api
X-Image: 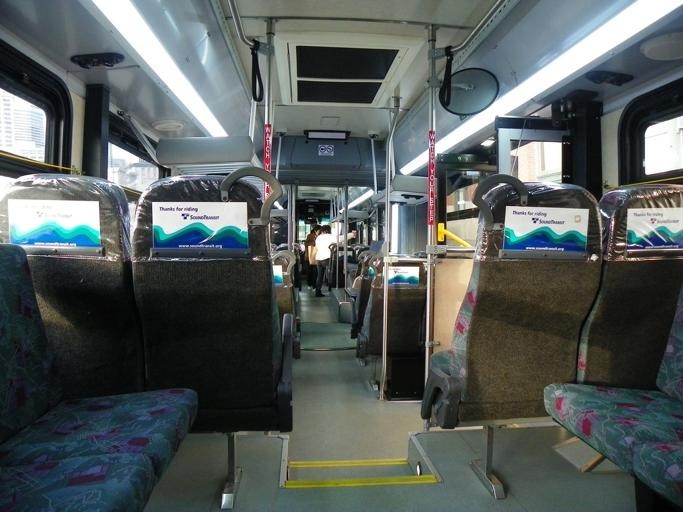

[308,246,318,265]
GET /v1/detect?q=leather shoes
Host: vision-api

[315,294,325,297]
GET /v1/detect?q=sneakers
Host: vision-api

[308,287,316,291]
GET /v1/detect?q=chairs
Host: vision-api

[321,174,682,512]
[0,174,302,510]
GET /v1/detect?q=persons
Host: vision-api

[305,224,355,297]
